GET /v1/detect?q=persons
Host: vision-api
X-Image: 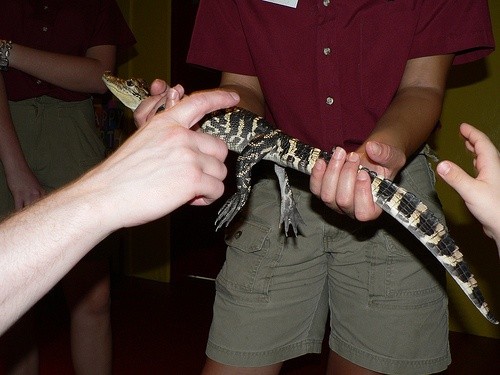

[136,1,495,375]
[437,121,500,254]
[0,89,240,338]
[0,0,135,375]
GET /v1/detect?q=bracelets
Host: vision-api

[0,41,11,68]
